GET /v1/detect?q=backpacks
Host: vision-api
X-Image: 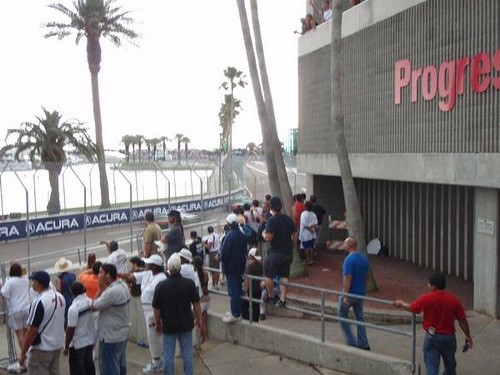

[204,233,215,253]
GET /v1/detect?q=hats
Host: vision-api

[248,247,262,260]
[154,239,166,248]
[177,247,193,262]
[167,252,181,270]
[144,254,163,266]
[225,213,239,225]
[54,257,73,272]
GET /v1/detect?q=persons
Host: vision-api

[349,0,360,8]
[63,283,97,375]
[308,0,333,23]
[92,263,130,375]
[242,247,263,322]
[0,211,220,361]
[294,14,319,36]
[298,201,318,265]
[116,253,167,374]
[261,196,298,309]
[337,238,371,351]
[19,271,66,375]
[215,212,247,323]
[391,273,473,375]
[215,192,326,321]
[151,256,202,375]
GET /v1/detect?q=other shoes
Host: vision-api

[263,296,275,305]
[8,361,28,373]
[221,311,243,323]
[275,299,286,308]
[142,358,163,374]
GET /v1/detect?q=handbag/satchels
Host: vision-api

[31,332,41,345]
